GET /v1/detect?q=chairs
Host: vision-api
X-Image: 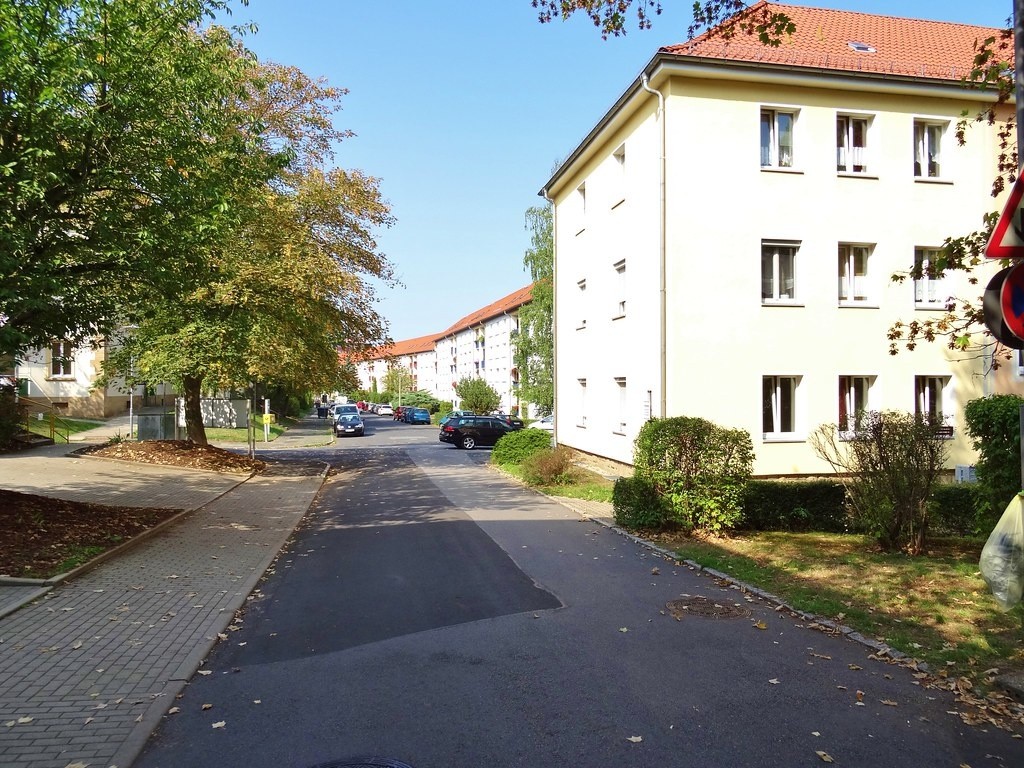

[351,416,359,421]
[340,417,347,421]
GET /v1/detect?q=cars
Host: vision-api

[393,406,431,425]
[527,415,554,435]
[356,401,393,416]
[334,413,365,437]
[329,407,335,425]
[438,416,526,450]
[491,414,525,429]
[437,411,475,430]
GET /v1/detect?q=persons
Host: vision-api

[328,400,335,406]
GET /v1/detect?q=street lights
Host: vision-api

[123,325,140,438]
[398,372,404,406]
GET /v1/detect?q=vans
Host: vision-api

[330,404,362,432]
